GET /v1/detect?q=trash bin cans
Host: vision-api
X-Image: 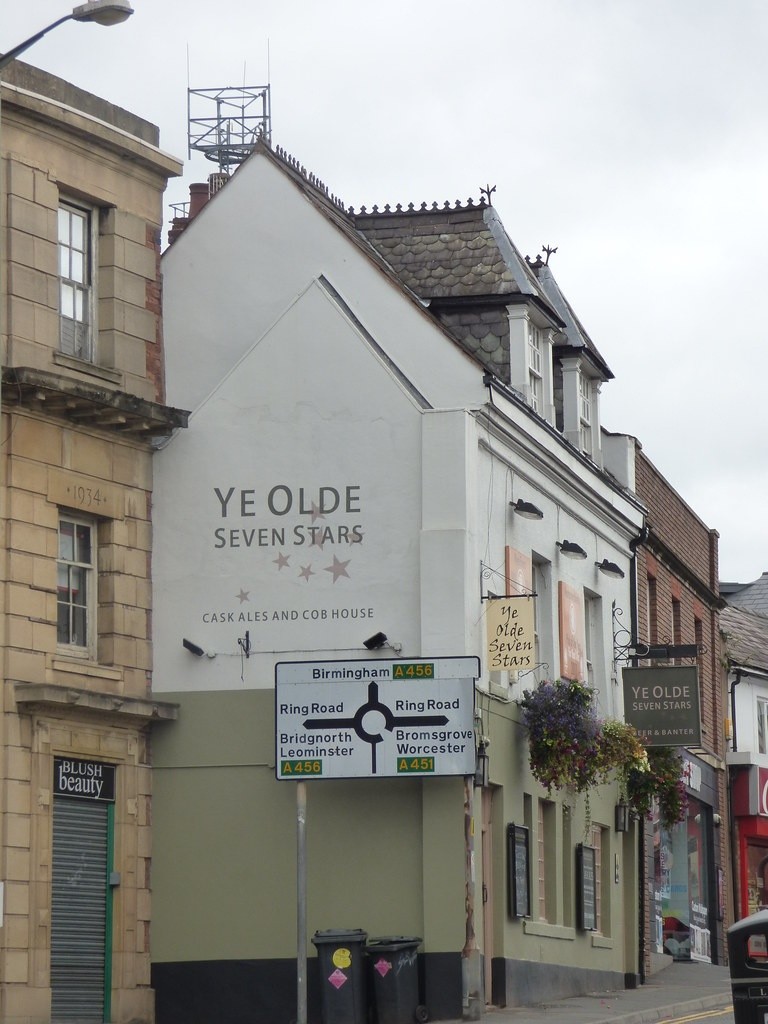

[367,936,429,1024]
[726,908,768,1024]
[310,928,368,1024]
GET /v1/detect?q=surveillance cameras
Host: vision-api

[362,632,388,651]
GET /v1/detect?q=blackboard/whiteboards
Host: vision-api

[578,842,599,931]
[506,822,533,919]
